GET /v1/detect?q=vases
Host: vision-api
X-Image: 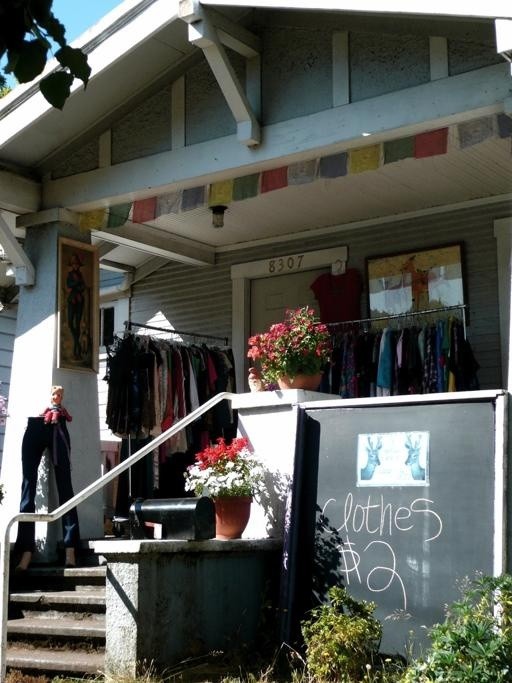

[278,370,324,390]
[213,496,253,539]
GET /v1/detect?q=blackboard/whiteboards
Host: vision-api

[300,386,508,671]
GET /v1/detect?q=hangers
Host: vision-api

[107,321,231,364]
[326,304,472,349]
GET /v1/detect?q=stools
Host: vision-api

[129,496,216,539]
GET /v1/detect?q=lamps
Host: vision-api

[209,206,228,227]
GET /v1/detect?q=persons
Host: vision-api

[66,255,86,360]
[39,385,73,425]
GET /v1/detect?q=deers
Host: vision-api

[361,436,383,480]
[404,434,425,481]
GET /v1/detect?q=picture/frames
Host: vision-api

[364,240,470,328]
[57,237,99,374]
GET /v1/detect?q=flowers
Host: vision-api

[183,437,265,500]
[247,305,333,392]
